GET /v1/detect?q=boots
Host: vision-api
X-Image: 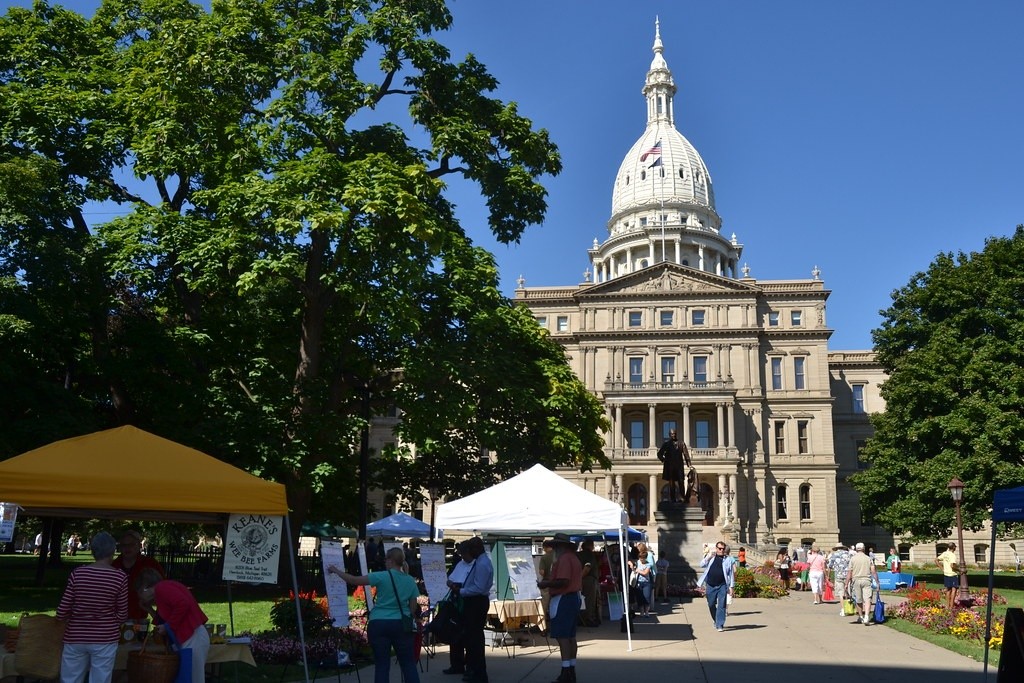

[549,666,576,683]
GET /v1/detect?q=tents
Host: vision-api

[299,511,444,540]
[983,485,1024,683]
[0,424,309,683]
[435,462,649,653]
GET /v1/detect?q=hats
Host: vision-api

[546,534,577,550]
[832,543,848,551]
[855,543,864,550]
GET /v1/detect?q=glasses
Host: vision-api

[717,546,725,550]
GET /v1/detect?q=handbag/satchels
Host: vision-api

[403,614,419,633]
[428,588,473,645]
[629,571,639,587]
[162,624,193,683]
[842,590,856,615]
[874,591,885,622]
[823,579,834,600]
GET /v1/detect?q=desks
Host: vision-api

[0,635,257,683]
[872,571,913,590]
[487,600,552,659]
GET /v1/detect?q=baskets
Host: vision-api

[127,630,180,683]
[14,613,66,679]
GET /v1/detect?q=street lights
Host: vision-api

[428,484,439,542]
[946,475,974,608]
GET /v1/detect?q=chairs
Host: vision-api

[394,607,436,676]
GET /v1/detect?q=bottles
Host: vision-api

[119,622,136,644]
[216,623,227,639]
[204,623,213,638]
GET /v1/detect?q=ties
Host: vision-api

[463,561,476,587]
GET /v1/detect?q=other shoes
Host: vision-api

[714,623,716,628]
[443,667,465,673]
[462,675,472,681]
[717,627,723,631]
[645,613,649,616]
[863,618,870,625]
[640,614,644,616]
[857,618,863,622]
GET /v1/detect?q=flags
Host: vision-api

[640,140,661,162]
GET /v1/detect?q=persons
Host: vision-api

[443,535,494,683]
[697,541,737,632]
[142,537,147,554]
[738,547,746,567]
[703,544,709,559]
[1014,553,1021,573]
[326,546,420,683]
[34,531,43,555]
[776,542,880,626]
[657,428,693,503]
[543,533,582,683]
[655,551,669,603]
[539,538,658,637]
[55,530,210,683]
[65,535,79,556]
[869,548,900,572]
[934,542,968,609]
